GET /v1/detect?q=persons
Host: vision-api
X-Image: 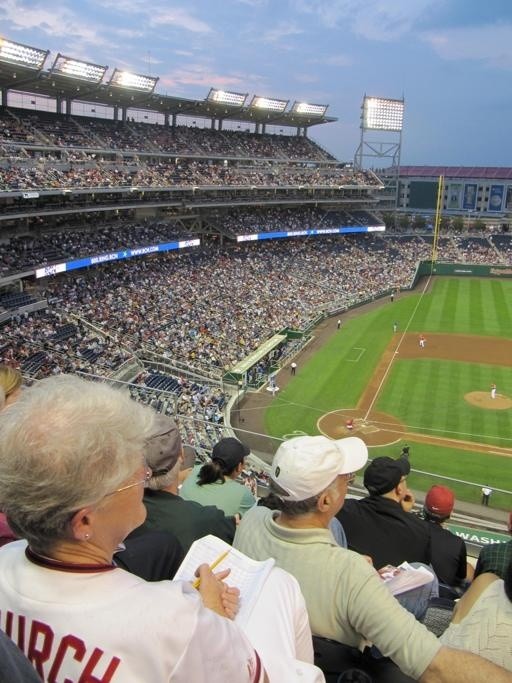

[391,293,394,301]
[400,444,410,458]
[0,273,416,461]
[233,436,512,683]
[338,456,431,571]
[291,362,297,375]
[337,319,340,329]
[481,485,492,505]
[346,419,353,429]
[394,323,397,332]
[475,512,511,577]
[421,486,475,599]
[491,384,496,399]
[129,407,237,553]
[419,335,426,348]
[437,558,512,670]
[113,518,183,581]
[0,102,512,275]
[0,375,324,682]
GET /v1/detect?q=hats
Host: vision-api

[144,414,181,477]
[364,456,410,495]
[268,436,369,502]
[426,485,454,515]
[212,439,250,474]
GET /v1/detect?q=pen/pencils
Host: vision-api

[191,547,231,588]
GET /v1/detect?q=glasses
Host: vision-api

[344,473,356,485]
[105,464,150,496]
[399,475,408,483]
